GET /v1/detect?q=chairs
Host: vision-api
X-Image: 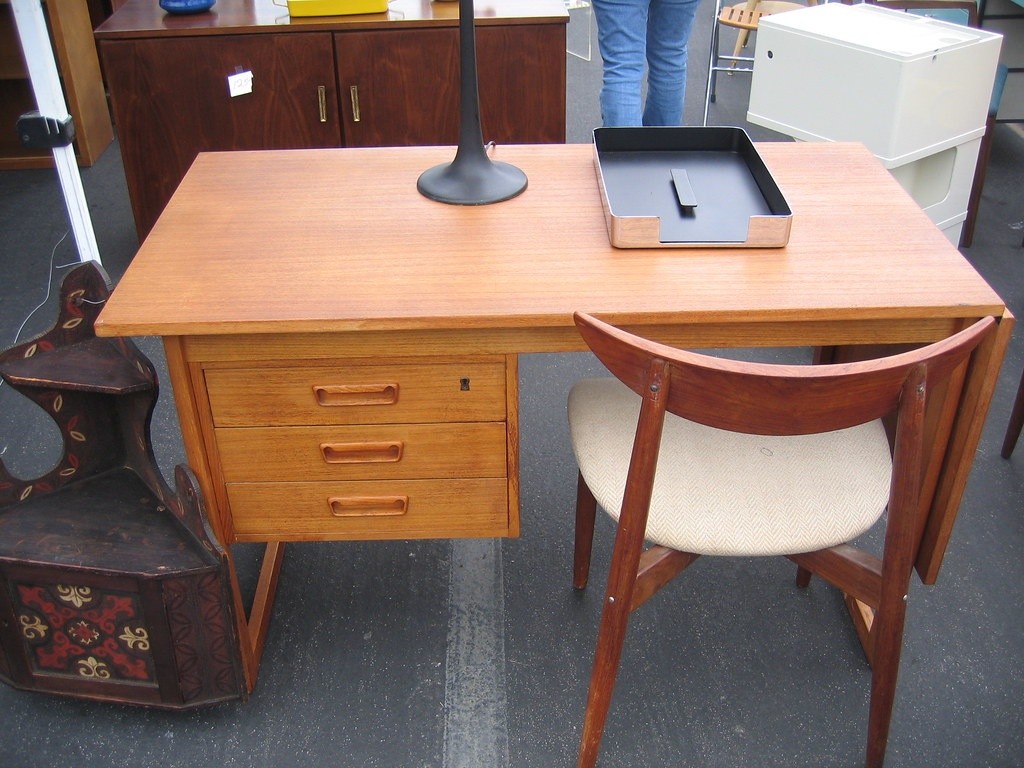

[840,0,1009,249]
[566,308,998,768]
[701,1,829,127]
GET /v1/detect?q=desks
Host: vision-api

[93,137,1016,695]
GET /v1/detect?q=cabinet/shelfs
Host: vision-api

[86,0,128,125]
[0,0,115,170]
[745,3,1004,249]
[93,0,571,249]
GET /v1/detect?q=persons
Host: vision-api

[591,0,702,127]
